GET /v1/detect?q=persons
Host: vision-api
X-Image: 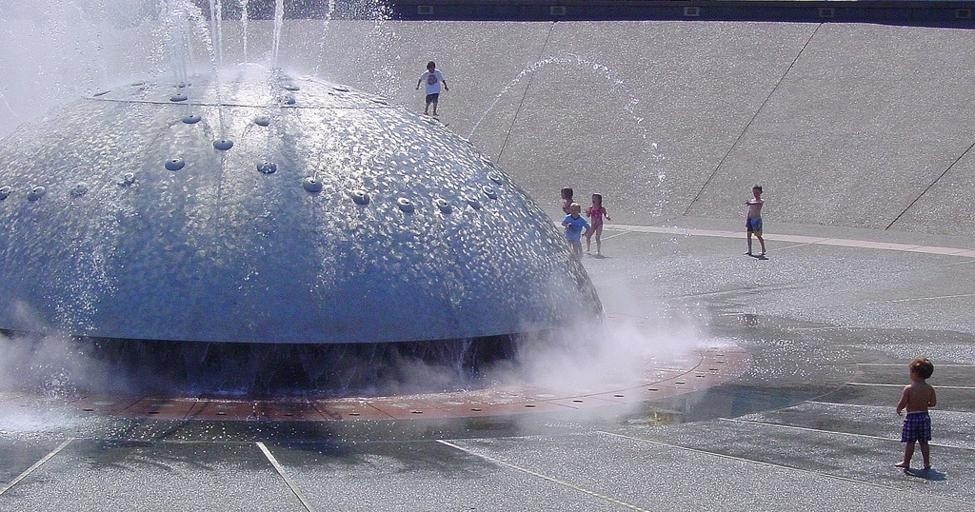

[743,186,766,256]
[415,61,449,116]
[894,358,936,469]
[561,203,592,256]
[560,186,583,258]
[585,193,610,254]
